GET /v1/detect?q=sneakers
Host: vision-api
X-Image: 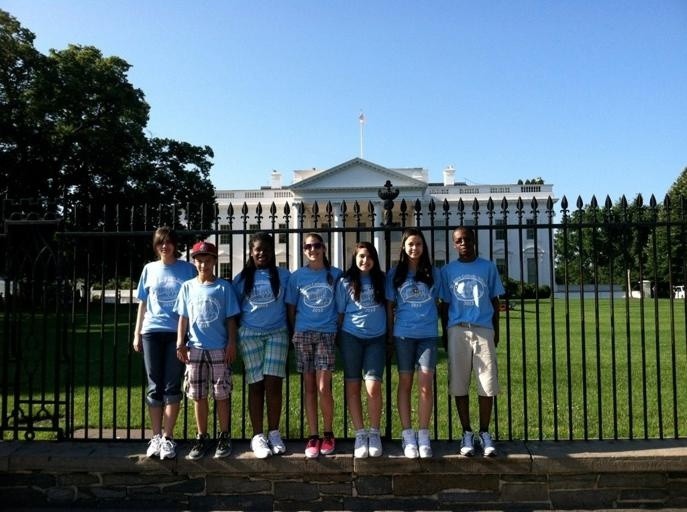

[147,434,178,459]
[478,431,496,457]
[189,432,210,459]
[355,430,382,459]
[251,428,286,458]
[214,432,232,457]
[401,429,434,459]
[305,435,335,458]
[461,429,475,457]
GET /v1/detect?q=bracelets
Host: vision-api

[176,345,187,351]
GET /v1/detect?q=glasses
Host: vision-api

[303,242,322,250]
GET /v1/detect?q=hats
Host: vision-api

[191,240,217,259]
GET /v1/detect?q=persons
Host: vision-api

[438,226,506,458]
[131,224,199,462]
[384,227,442,460]
[284,233,345,459]
[230,231,294,459]
[175,241,240,461]
[335,240,388,460]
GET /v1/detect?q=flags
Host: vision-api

[358,110,366,126]
